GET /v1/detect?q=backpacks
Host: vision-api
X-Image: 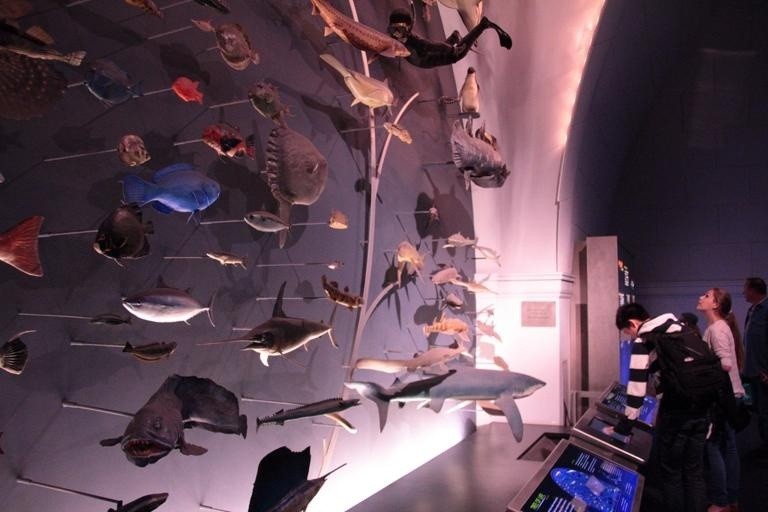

[639,319,723,410]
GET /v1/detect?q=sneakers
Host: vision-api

[706,504,729,512]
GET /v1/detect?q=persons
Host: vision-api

[678,312,702,339]
[739,277,767,461]
[604,301,710,512]
[696,287,747,512]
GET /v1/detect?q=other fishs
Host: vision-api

[0,0,547,468]
[247,446,348,512]
[107,493,168,512]
